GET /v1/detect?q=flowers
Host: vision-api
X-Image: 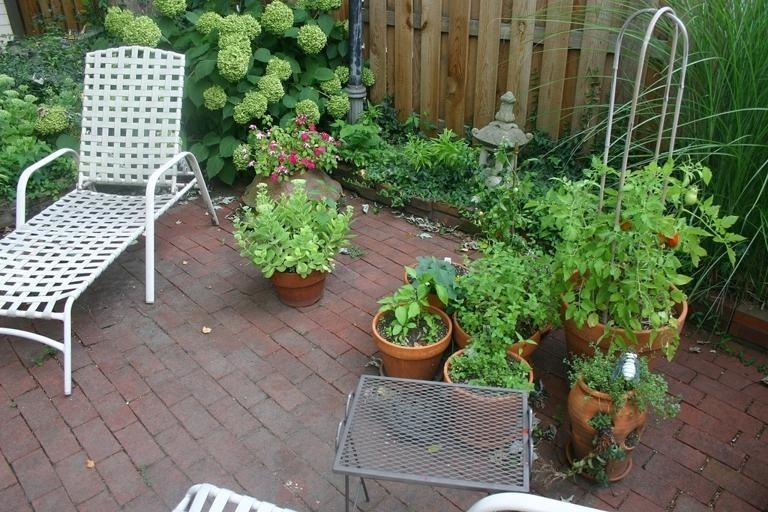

[238,111,340,179]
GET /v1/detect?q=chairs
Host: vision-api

[0,47,219,399]
[168,477,616,512]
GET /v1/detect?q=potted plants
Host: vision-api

[226,172,357,308]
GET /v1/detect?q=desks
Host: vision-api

[330,373,532,512]
